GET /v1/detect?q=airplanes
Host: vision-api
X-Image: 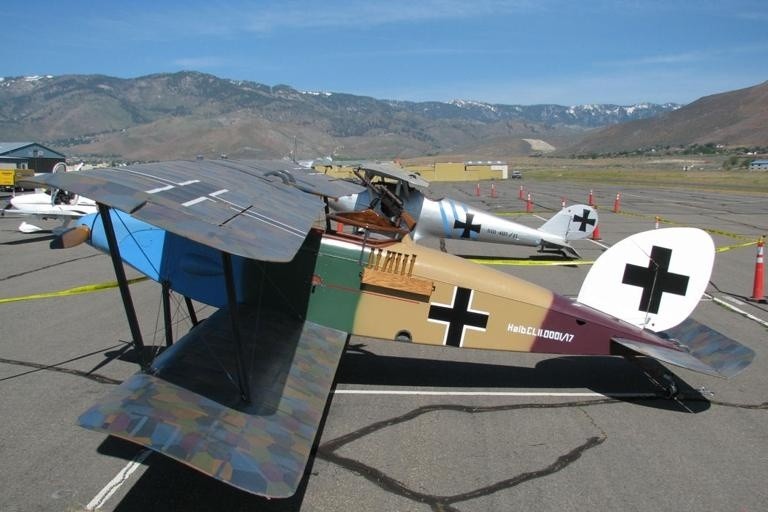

[0,148,762,501]
[317,156,601,265]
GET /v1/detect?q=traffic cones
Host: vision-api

[474,167,623,243]
[748,236,766,301]
